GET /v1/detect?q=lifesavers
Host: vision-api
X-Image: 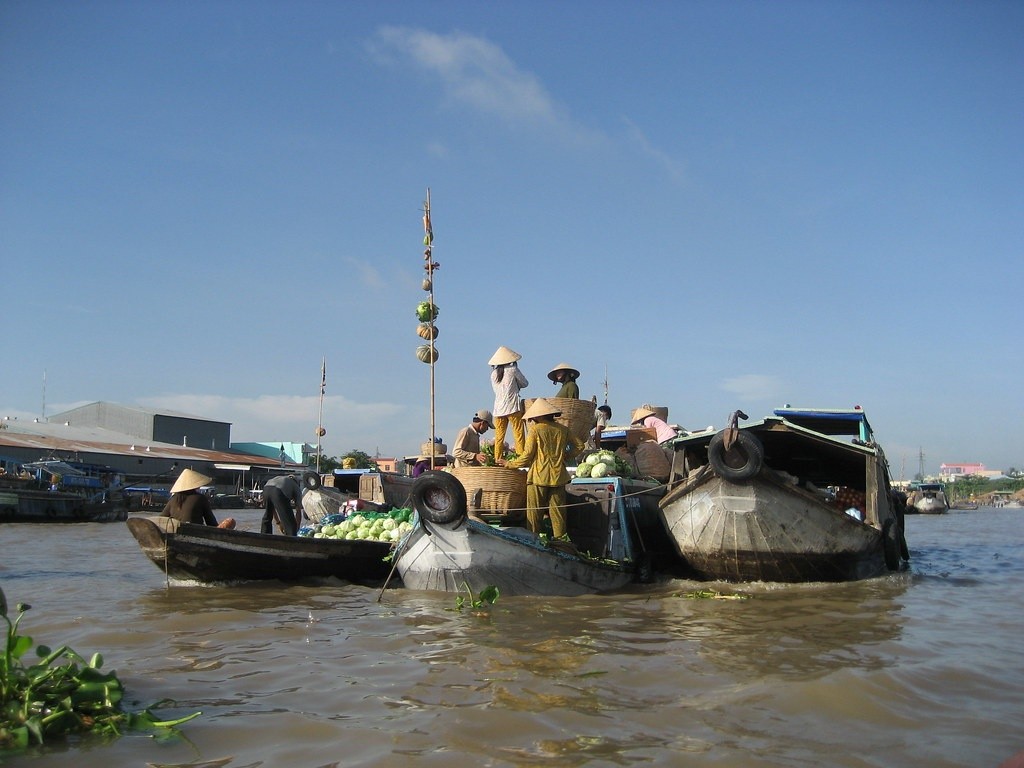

[707,429,766,484]
[303,471,322,490]
[410,469,468,524]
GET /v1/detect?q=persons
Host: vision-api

[261,475,302,536]
[158,469,236,530]
[547,363,580,399]
[496,397,585,541]
[584,405,612,449]
[631,408,677,447]
[413,456,431,478]
[452,410,496,468]
[488,346,529,466]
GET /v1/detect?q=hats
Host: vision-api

[474,410,496,430]
[416,456,429,462]
[488,346,522,365]
[521,398,562,419]
[631,408,657,425]
[170,468,212,493]
[547,363,580,381]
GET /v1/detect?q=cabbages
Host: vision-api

[575,453,618,478]
[313,514,414,542]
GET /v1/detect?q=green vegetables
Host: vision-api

[479,444,518,467]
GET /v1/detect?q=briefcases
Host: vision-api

[625,427,658,449]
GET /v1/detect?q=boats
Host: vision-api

[910,482,949,514]
[954,504,978,509]
[126,452,455,583]
[657,405,910,583]
[377,422,690,603]
[124,480,308,512]
[0,457,129,522]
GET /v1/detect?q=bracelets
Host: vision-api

[276,520,281,525]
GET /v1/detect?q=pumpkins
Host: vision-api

[417,322,439,340]
[415,302,440,323]
[422,279,431,291]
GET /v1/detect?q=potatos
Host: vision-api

[416,344,443,365]
[833,487,865,512]
